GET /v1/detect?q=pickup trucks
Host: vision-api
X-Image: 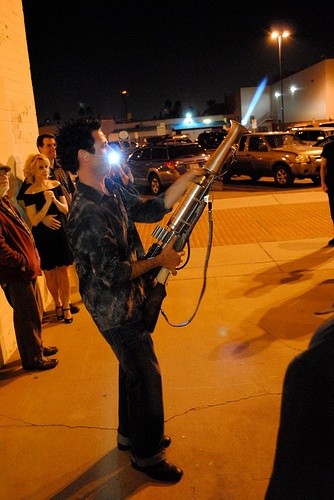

[203,132,326,188]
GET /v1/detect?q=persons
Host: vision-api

[105,143,134,190]
[263,310,334,500]
[318,136,334,248]
[16,133,80,315]
[22,153,75,324]
[53,113,208,462]
[0,163,59,371]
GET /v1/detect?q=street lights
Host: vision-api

[268,28,292,126]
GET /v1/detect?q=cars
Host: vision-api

[285,121,334,146]
[108,139,140,158]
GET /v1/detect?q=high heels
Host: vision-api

[55,305,64,320]
[62,307,73,323]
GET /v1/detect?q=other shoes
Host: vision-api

[329,239,334,246]
[69,304,80,314]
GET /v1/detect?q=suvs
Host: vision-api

[127,136,215,197]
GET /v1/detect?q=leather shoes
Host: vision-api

[117,432,171,452]
[23,358,59,371]
[131,451,183,483]
[43,345,58,356]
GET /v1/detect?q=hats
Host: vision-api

[0,163,11,172]
